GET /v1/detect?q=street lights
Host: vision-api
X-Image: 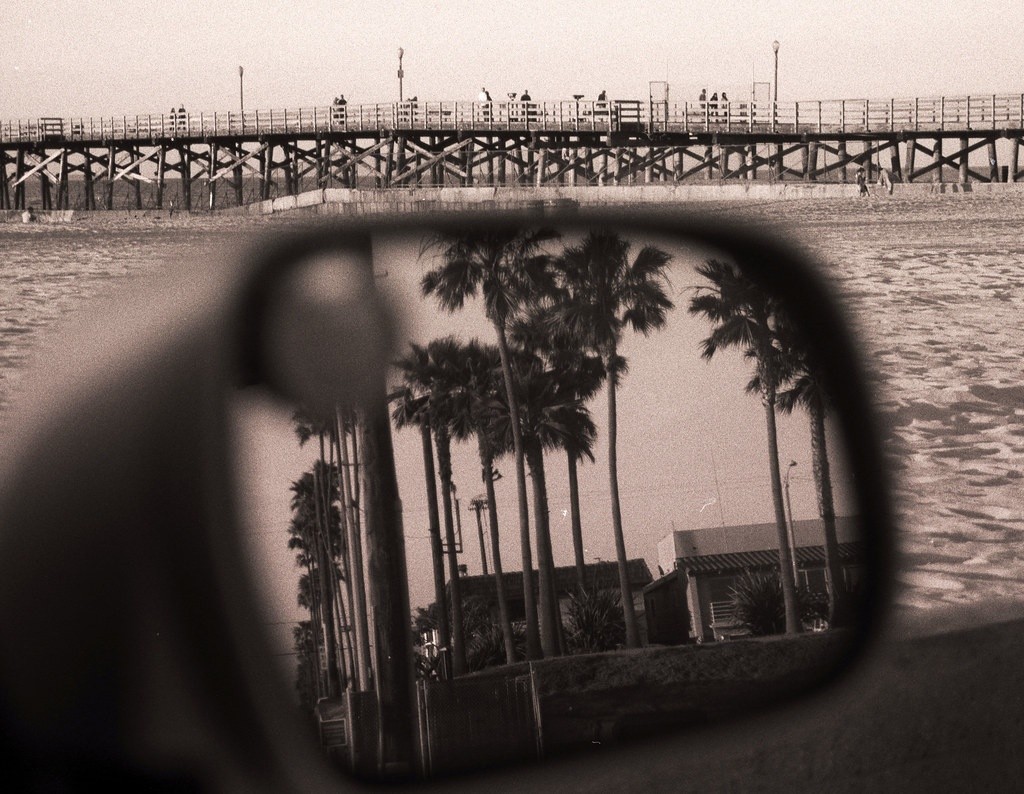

[784,457,799,593]
[239,65,247,123]
[773,38,783,126]
[397,46,406,102]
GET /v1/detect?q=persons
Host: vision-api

[170,104,186,130]
[22,207,36,224]
[855,168,870,198]
[597,90,608,121]
[700,89,729,122]
[332,94,348,125]
[404,87,531,121]
